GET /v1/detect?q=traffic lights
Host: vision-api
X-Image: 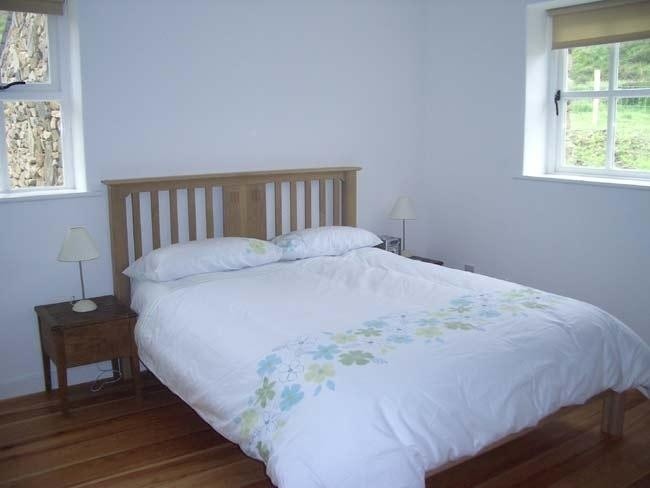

[120,225,385,283]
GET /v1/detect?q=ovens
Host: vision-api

[99,166,648,488]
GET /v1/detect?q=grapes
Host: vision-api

[31,295,139,407]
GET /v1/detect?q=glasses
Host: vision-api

[388,192,417,258]
[55,225,103,313]
